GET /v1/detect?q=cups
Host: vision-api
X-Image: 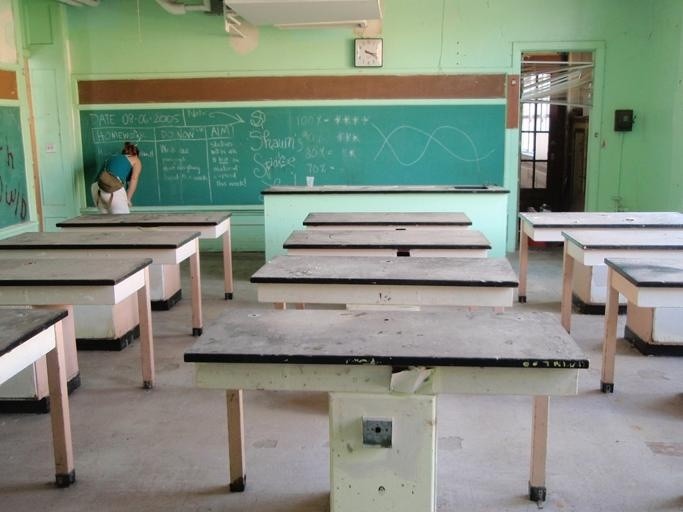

[306,176,314,186]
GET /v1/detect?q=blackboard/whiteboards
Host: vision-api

[73,98,508,216]
[0,99,39,240]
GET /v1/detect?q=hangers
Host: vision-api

[354,37,382,68]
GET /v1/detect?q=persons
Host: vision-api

[89,141,141,214]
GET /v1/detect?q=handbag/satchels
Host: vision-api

[96,166,123,193]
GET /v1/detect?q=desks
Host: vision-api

[0,231,203,336]
[514,208,683,396]
[1,309,76,488]
[183,309,590,501]
[250,255,520,309]
[1,258,158,389]
[302,212,472,229]
[282,229,492,259]
[56,211,234,300]
